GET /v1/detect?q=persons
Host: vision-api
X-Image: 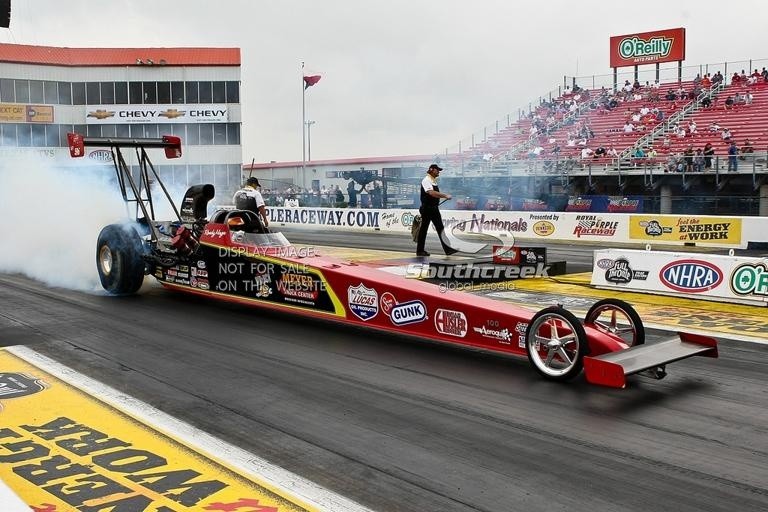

[233,179,389,225]
[416,165,458,255]
[520,68,768,170]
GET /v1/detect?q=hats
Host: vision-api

[429,164,443,171]
[247,177,261,187]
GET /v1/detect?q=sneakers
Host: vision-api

[417,251,430,256]
[446,249,458,254]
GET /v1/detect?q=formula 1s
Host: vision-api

[62,131,719,391]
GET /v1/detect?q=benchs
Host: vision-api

[456,77,767,162]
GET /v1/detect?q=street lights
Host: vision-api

[304,120,315,161]
